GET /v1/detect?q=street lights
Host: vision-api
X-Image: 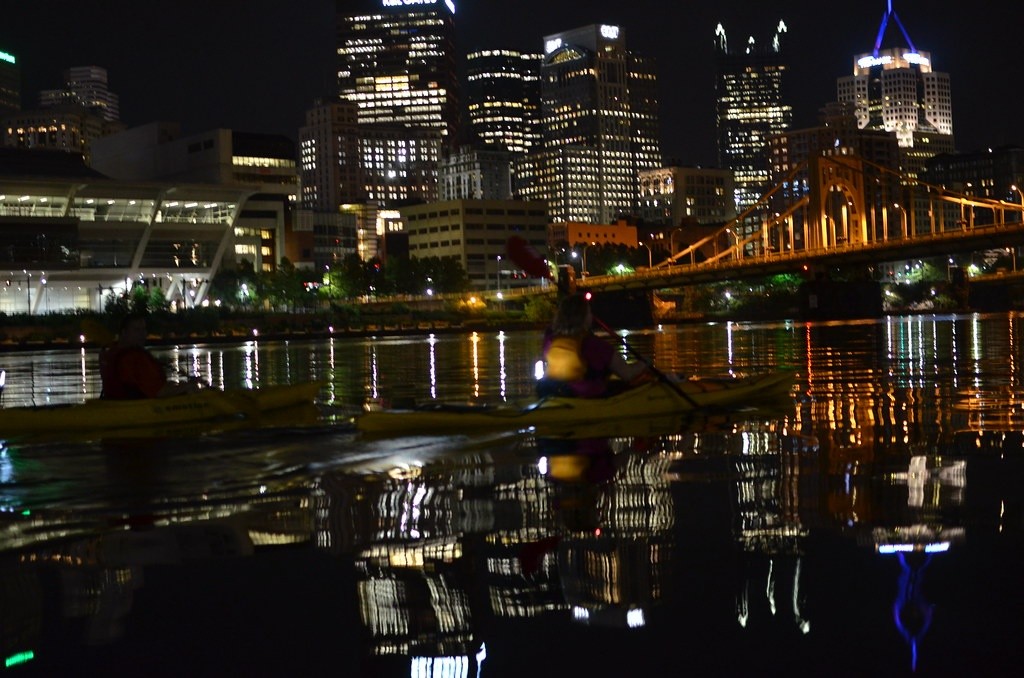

[11,246,590,319]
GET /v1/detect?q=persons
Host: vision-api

[99,314,204,399]
[542,294,638,399]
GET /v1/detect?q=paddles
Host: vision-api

[505,235,702,410]
[143,347,208,387]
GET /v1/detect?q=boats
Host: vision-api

[360,370,800,446]
[1,373,325,447]
